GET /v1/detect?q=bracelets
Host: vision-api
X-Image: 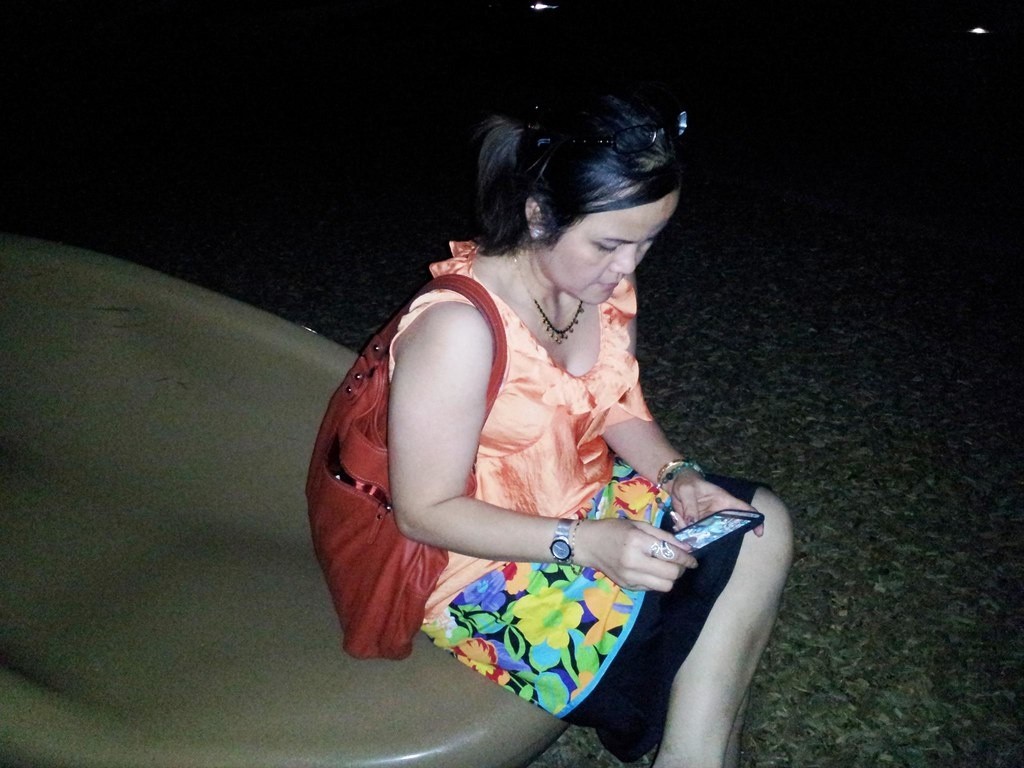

[655,459,707,515]
[571,521,583,575]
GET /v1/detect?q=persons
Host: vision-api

[387,84,795,768]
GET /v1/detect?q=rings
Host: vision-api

[648,540,673,559]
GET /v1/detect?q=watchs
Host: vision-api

[549,518,574,564]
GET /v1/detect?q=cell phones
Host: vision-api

[674,508,765,558]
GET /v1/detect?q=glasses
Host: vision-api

[537,110,688,156]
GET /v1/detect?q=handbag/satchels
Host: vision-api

[304,274,508,660]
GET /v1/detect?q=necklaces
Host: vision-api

[512,254,585,344]
